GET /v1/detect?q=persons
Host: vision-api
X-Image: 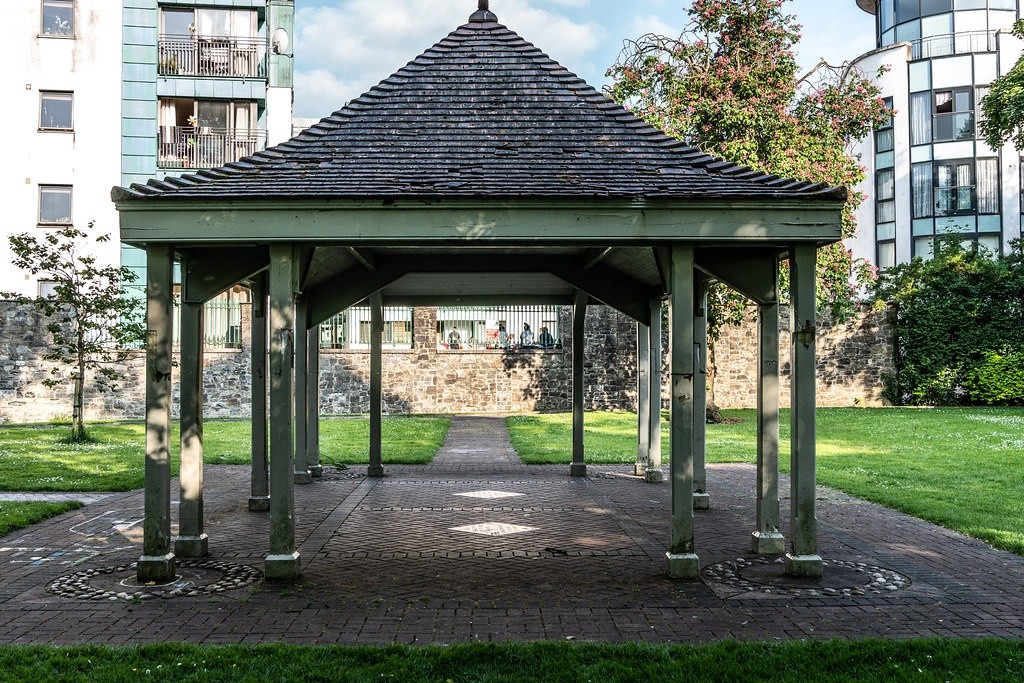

[539,327,553,347]
[498,323,515,349]
[520,322,534,348]
[448,326,461,349]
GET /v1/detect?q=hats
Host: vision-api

[524,323,530,329]
[499,324,505,331]
[540,327,548,331]
